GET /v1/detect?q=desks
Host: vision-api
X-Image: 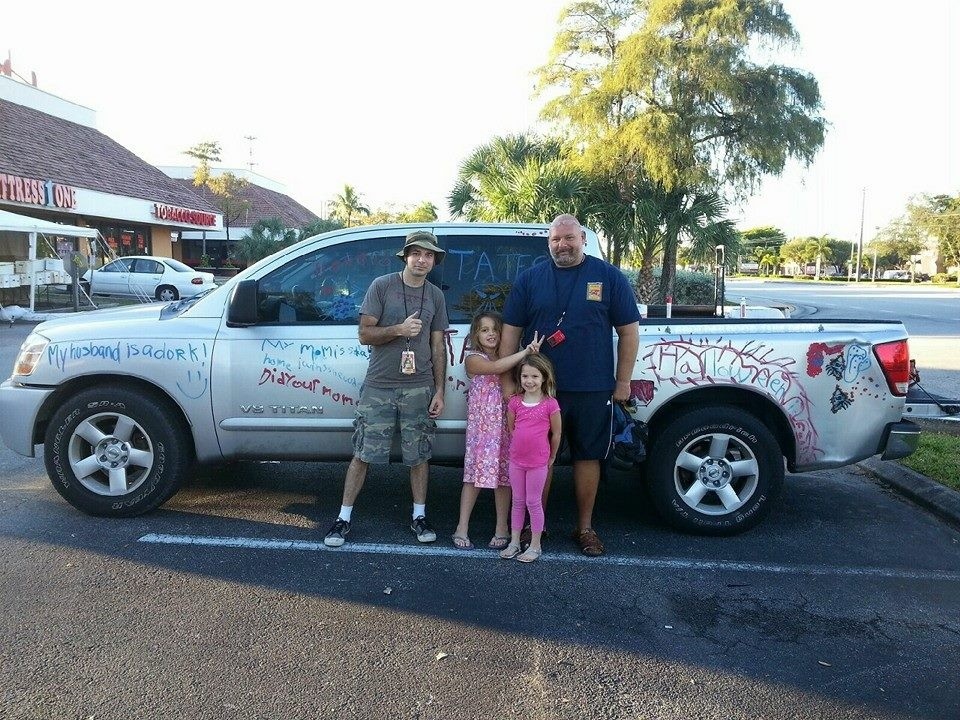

[0,280,91,312]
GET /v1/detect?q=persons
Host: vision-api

[324,231,451,546]
[498,212,641,557]
[501,353,562,564]
[451,313,546,551]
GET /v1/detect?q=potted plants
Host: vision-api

[193,253,217,275]
[218,252,240,277]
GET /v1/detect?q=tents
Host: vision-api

[0,209,99,314]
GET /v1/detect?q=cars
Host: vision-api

[57,255,216,301]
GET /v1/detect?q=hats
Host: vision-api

[395,230,447,265]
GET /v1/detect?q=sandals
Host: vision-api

[515,548,541,563]
[499,544,523,559]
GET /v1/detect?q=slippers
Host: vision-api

[488,535,511,549]
[451,533,475,550]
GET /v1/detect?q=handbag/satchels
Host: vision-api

[607,395,651,469]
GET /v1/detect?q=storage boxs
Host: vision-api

[0,256,73,289]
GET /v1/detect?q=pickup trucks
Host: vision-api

[1,221,921,534]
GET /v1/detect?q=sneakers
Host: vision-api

[519,523,545,551]
[324,517,352,546]
[410,515,436,543]
[574,526,605,556]
